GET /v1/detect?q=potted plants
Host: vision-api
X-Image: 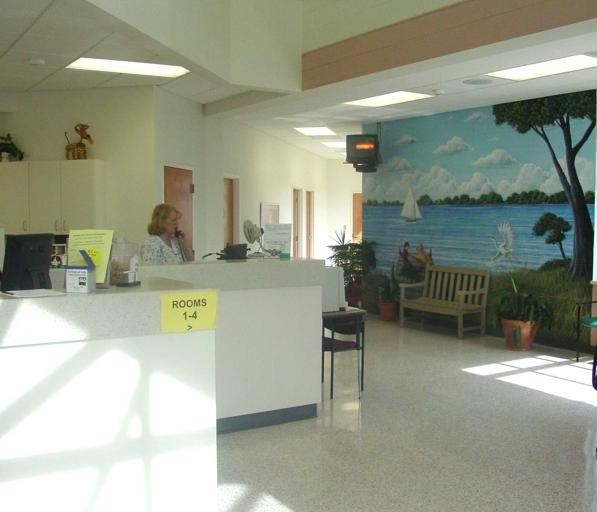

[325,228,397,322]
[497,293,551,351]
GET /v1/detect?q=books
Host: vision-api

[0,229,116,303]
[264,224,292,259]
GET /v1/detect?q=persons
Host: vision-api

[413,244,432,265]
[140,204,193,263]
[399,242,409,263]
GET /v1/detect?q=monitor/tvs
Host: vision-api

[1,233,53,291]
[228,244,247,258]
[347,135,378,162]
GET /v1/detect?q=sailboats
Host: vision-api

[399,187,422,224]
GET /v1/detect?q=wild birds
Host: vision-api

[475,221,514,267]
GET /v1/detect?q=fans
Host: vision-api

[242,219,265,258]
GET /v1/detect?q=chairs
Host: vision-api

[322,310,365,401]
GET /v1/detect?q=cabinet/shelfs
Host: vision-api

[0,161,29,234]
[28,160,94,234]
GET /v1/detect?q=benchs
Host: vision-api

[398,264,490,339]
